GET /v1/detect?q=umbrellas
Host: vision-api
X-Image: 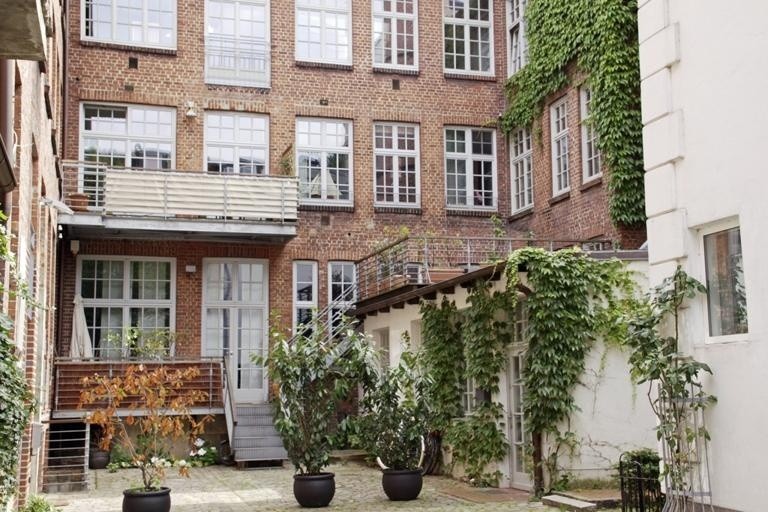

[301,166,340,197]
[71,294,95,362]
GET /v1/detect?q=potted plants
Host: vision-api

[344,359,447,503]
[71,320,217,511]
[247,299,387,507]
[90,449,110,470]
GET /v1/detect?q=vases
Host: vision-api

[67,193,90,212]
